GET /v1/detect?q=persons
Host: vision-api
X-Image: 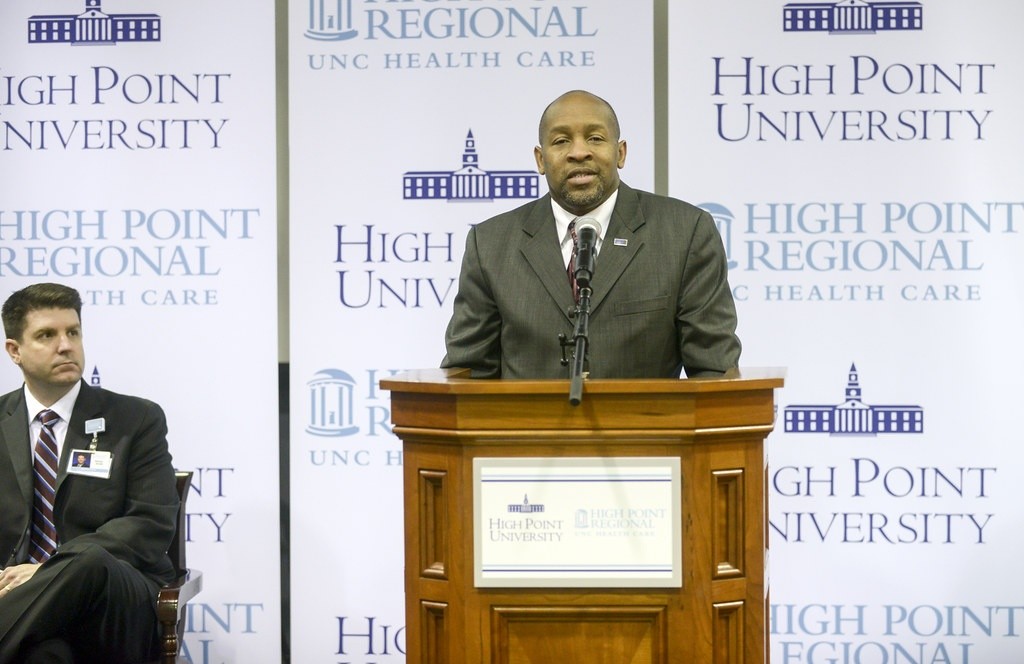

[0,283,180,664]
[439,89,742,380]
[72,453,89,467]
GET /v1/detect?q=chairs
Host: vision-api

[157,471,205,664]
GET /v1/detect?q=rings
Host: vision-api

[5,586,11,591]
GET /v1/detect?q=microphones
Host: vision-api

[573,217,602,288]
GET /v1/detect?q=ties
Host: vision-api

[27,412,61,565]
[566,220,595,303]
